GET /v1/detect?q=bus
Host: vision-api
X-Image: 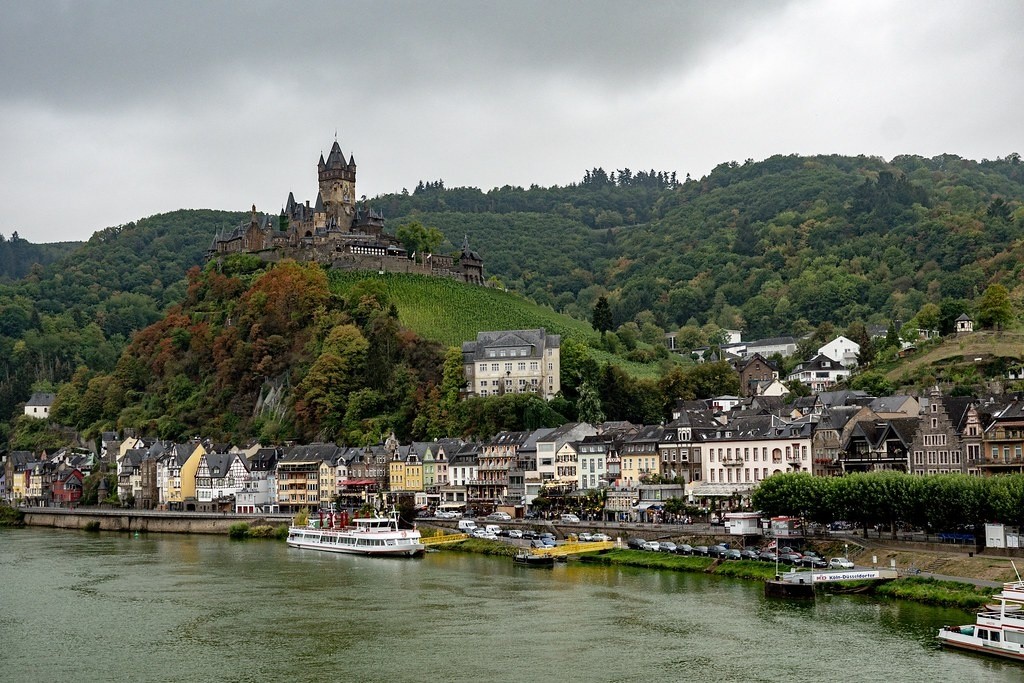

[433,506,463,519]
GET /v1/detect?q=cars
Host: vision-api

[486,511,512,521]
[536,533,557,542]
[466,524,511,540]
[508,529,523,539]
[591,534,613,541]
[828,556,855,569]
[522,530,540,541]
[692,546,829,569]
[564,533,579,540]
[676,544,694,555]
[627,539,646,550]
[642,541,660,552]
[541,537,558,548]
[659,542,677,553]
[531,540,546,548]
[579,533,594,542]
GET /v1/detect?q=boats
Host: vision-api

[932,559,1024,661]
[516,552,554,564]
[285,503,428,559]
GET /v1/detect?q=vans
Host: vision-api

[458,520,478,532]
[560,514,581,524]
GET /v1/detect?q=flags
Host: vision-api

[768,540,776,549]
